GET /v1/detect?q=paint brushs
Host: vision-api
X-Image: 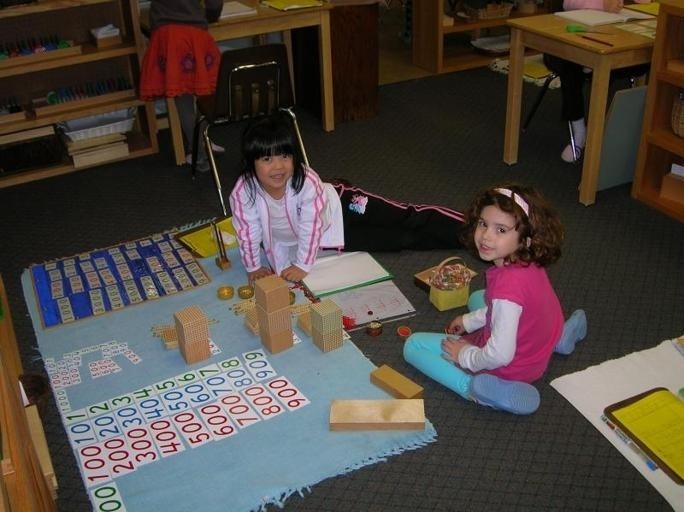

[575,32,614,47]
[209,221,228,263]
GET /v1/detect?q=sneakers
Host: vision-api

[469,374,540,415]
[555,309,588,355]
[562,131,583,163]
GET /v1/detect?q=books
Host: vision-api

[553,6,657,27]
[67,134,132,168]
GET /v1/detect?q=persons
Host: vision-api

[543,0,642,165]
[134,1,223,175]
[398,183,593,416]
[228,114,469,287]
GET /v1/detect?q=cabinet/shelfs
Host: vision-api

[625,0,683,223]
[407,1,555,80]
[0,1,160,191]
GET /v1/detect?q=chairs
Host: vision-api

[193,39,312,217]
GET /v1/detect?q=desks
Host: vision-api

[137,0,339,167]
[494,5,665,213]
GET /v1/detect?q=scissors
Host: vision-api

[565,24,599,33]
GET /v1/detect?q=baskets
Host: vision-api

[57,107,138,142]
